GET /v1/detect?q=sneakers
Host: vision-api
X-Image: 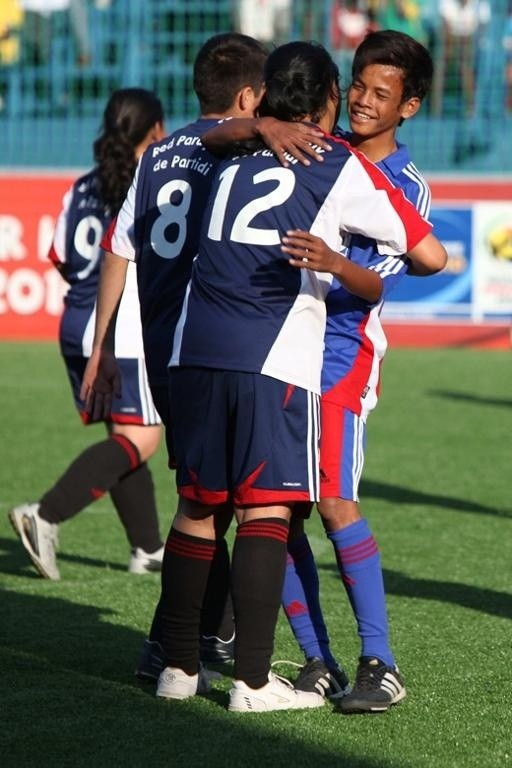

[8,503,61,581]
[134,633,407,713]
[128,543,165,574]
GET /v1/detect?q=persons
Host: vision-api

[8,86,166,583]
[239,0,511,137]
[76,26,450,712]
